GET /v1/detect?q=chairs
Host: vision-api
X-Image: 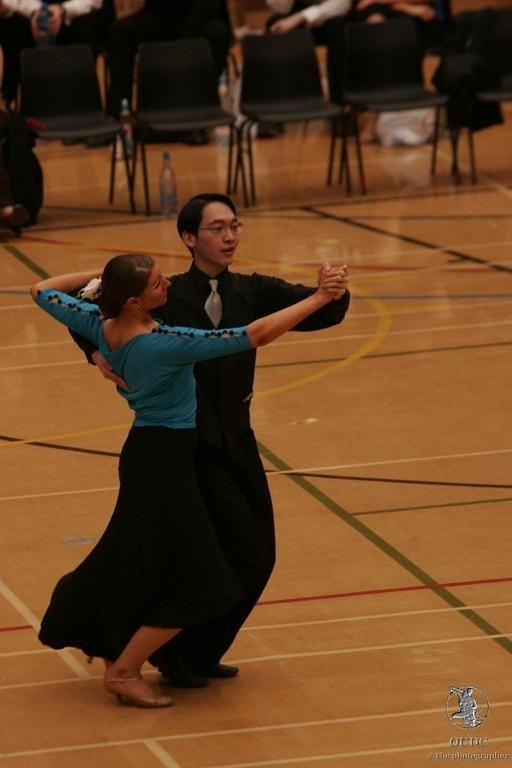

[17,8,509,223]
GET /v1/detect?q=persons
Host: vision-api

[2,0,512,149]
[25,251,350,709]
[66,189,350,691]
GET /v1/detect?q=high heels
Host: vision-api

[86,655,173,708]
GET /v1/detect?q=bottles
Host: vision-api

[119,98,137,160]
[36,3,52,33]
[159,153,177,215]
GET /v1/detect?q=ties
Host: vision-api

[203,278,224,328]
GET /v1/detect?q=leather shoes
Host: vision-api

[148,652,211,688]
[195,659,239,680]
[61,132,113,149]
[180,129,209,147]
[358,118,378,145]
[256,123,283,140]
